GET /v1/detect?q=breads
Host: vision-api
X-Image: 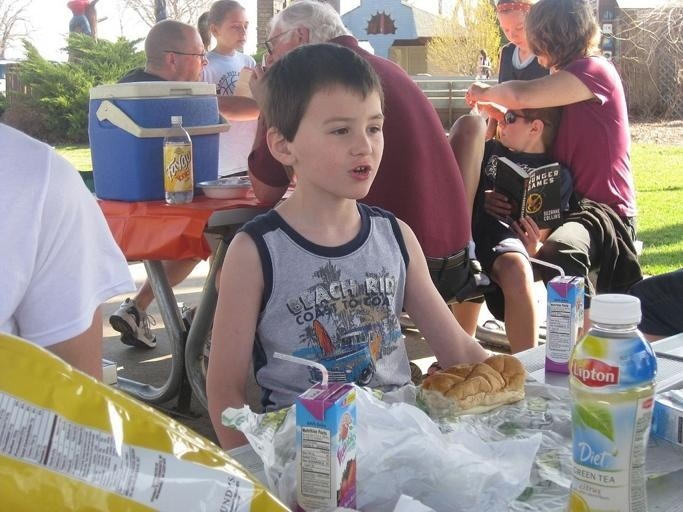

[419,355,526,417]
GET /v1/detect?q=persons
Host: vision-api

[497,2,547,82]
[471,108,570,354]
[205,44,494,451]
[465,1,641,307]
[247,0,469,299]
[109,19,260,350]
[476,47,491,83]
[198,0,257,98]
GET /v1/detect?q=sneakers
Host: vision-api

[179,308,211,360]
[110,299,157,349]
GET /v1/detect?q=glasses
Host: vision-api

[504,110,547,126]
[264,27,299,52]
[231,22,250,34]
[163,48,207,62]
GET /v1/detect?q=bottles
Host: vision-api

[650,389,682,447]
[162,115,194,205]
[567,293,658,512]
[295,381,357,512]
[545,275,585,375]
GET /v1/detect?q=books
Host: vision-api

[496,156,564,233]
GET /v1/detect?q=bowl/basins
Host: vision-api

[196,179,251,199]
